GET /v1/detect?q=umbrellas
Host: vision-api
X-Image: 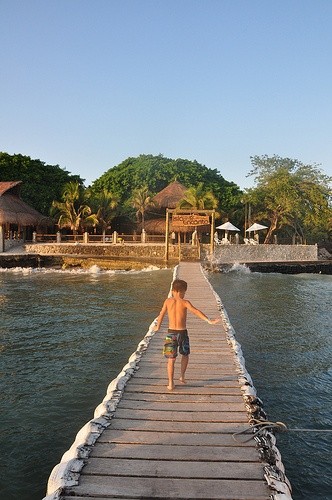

[216,222,240,238]
[246,223,268,234]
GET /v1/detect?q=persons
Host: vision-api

[214,231,218,241]
[192,230,197,246]
[152,280,221,390]
[171,231,175,244]
